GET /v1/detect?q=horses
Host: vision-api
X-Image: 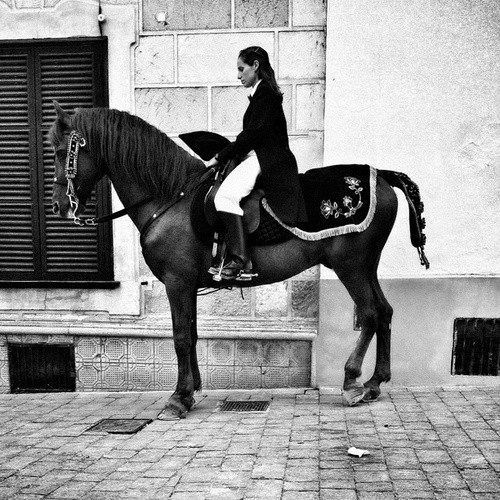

[46,97,430,422]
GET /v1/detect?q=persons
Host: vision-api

[207,46,298,280]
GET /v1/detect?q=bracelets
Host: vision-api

[215,153,220,161]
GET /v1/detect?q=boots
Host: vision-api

[208,211,255,279]
